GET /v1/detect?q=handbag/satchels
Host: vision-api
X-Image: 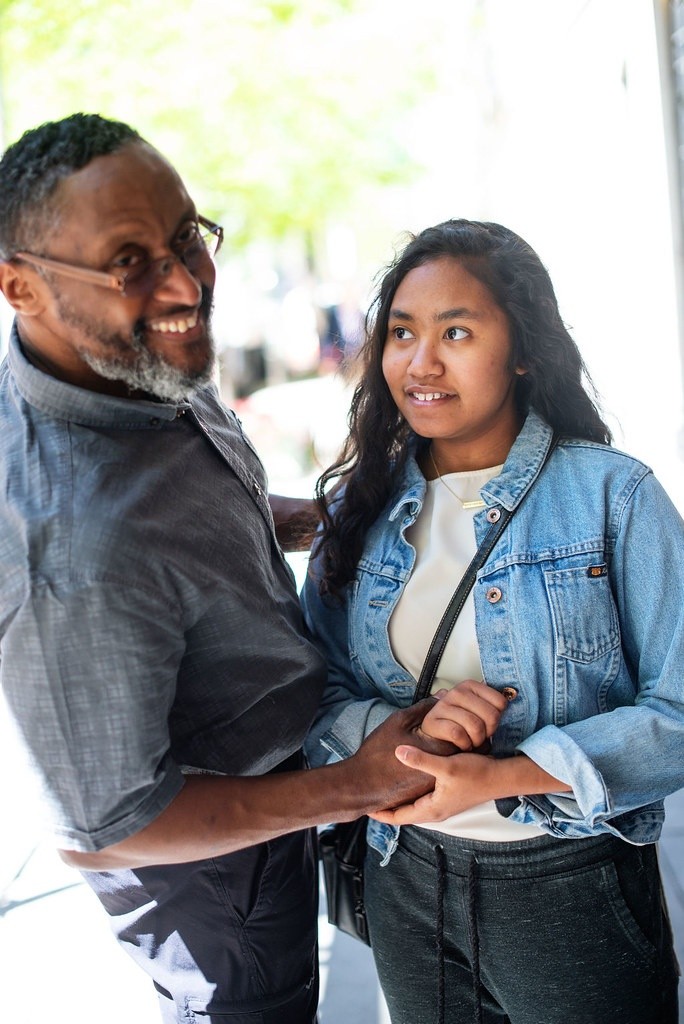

[319,815,371,949]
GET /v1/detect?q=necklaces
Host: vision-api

[428,442,487,510]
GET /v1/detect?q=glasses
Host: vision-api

[14,214,223,298]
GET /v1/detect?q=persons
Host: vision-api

[301,218,684,1024]
[0,114,458,1024]
